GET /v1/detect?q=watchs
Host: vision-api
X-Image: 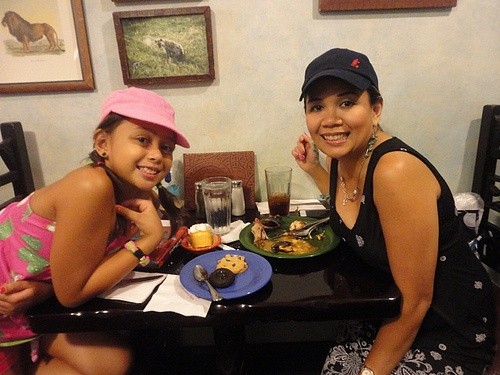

[359,362,375,375]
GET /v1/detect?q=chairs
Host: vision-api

[440,104,500,303]
[0,120,36,212]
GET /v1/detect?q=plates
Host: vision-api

[179,249,272,300]
[238,215,340,259]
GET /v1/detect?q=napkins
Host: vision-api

[94,271,167,304]
[144,274,212,319]
[256,200,297,216]
[215,220,250,244]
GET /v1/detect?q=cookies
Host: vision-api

[216,254,249,274]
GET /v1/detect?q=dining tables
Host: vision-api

[28,198,401,375]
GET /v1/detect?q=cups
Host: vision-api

[201,176,231,235]
[265,166,292,217]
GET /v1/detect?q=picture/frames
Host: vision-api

[112,5,215,88]
[0,0,97,96]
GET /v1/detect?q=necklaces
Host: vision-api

[337,126,380,205]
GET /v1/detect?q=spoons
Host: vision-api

[298,217,330,236]
[192,264,224,301]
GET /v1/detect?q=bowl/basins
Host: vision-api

[180,233,222,254]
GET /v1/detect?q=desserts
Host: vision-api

[187,223,214,248]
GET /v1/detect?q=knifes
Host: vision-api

[119,274,164,286]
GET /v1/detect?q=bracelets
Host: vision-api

[123,241,150,267]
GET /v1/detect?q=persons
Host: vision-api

[0,87,193,375]
[289,47,500,375]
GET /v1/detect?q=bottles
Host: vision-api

[195,181,206,220]
[231,179,245,216]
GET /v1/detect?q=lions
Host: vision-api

[1,10,65,53]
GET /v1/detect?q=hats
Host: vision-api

[299,49,379,101]
[99,87,191,149]
